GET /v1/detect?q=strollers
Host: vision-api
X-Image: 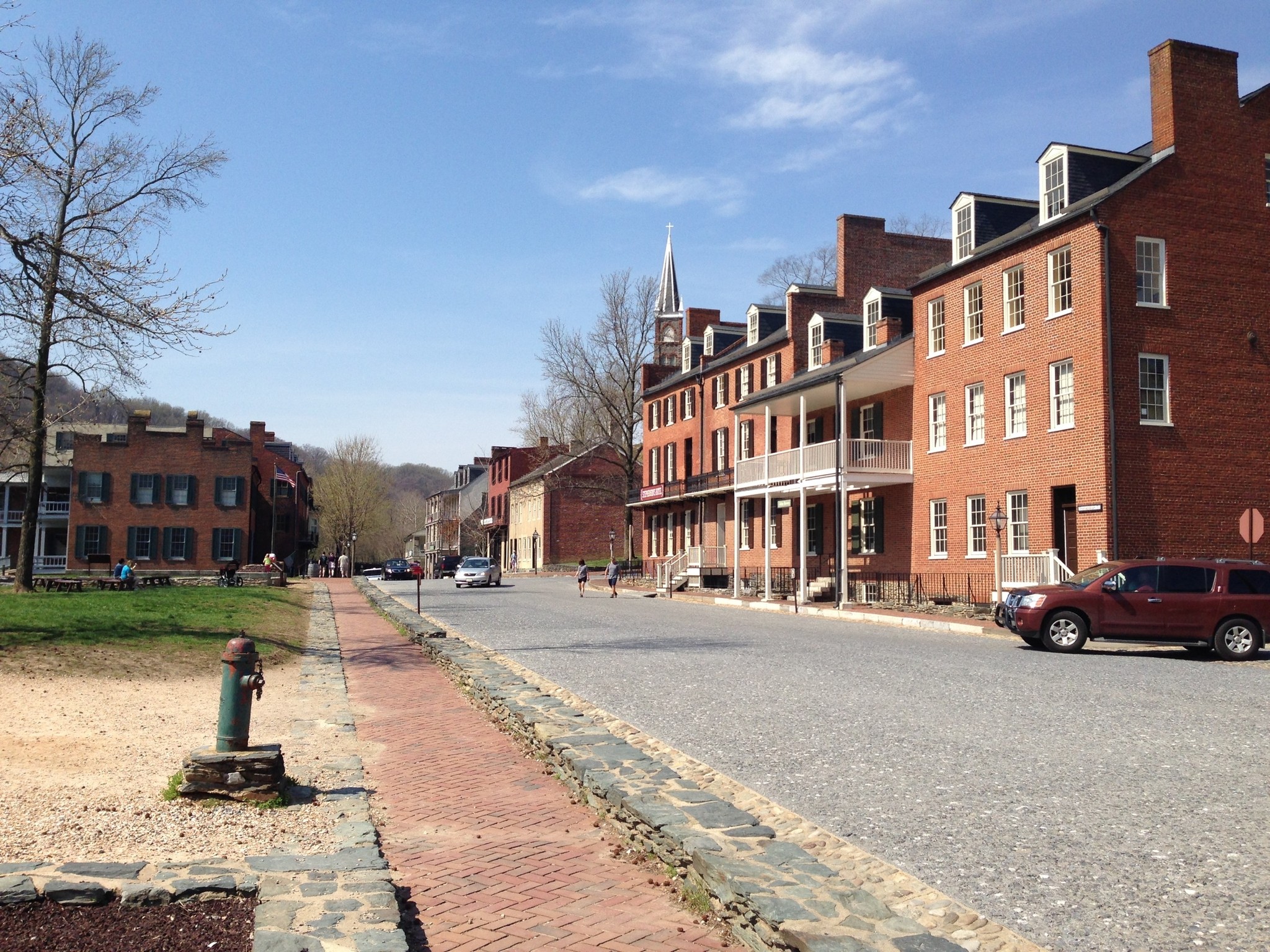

[218,558,243,588]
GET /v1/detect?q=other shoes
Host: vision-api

[580,593,583,597]
[610,594,614,598]
[615,592,618,597]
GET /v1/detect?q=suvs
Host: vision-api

[456,556,481,572]
[433,554,462,579]
[992,557,1270,662]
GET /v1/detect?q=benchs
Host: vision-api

[32,577,64,592]
[100,580,137,592]
[54,579,83,593]
[76,577,113,589]
[139,575,172,587]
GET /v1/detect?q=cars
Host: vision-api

[453,557,503,589]
[409,562,424,580]
[363,567,382,580]
[380,558,414,581]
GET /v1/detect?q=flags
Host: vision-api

[276,465,296,488]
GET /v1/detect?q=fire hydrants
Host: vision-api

[213,628,265,754]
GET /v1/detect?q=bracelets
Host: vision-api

[135,564,137,566]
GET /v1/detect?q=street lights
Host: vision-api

[989,506,1009,609]
[532,530,539,574]
[608,529,616,563]
[350,530,357,578]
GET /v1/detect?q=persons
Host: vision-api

[393,563,397,566]
[121,560,141,591]
[390,562,393,566]
[338,553,349,578]
[328,552,336,577]
[574,558,590,597]
[482,561,487,566]
[399,561,405,566]
[114,558,137,589]
[263,554,275,572]
[1117,571,1155,592]
[605,556,621,598]
[510,550,518,573]
[318,552,327,577]
